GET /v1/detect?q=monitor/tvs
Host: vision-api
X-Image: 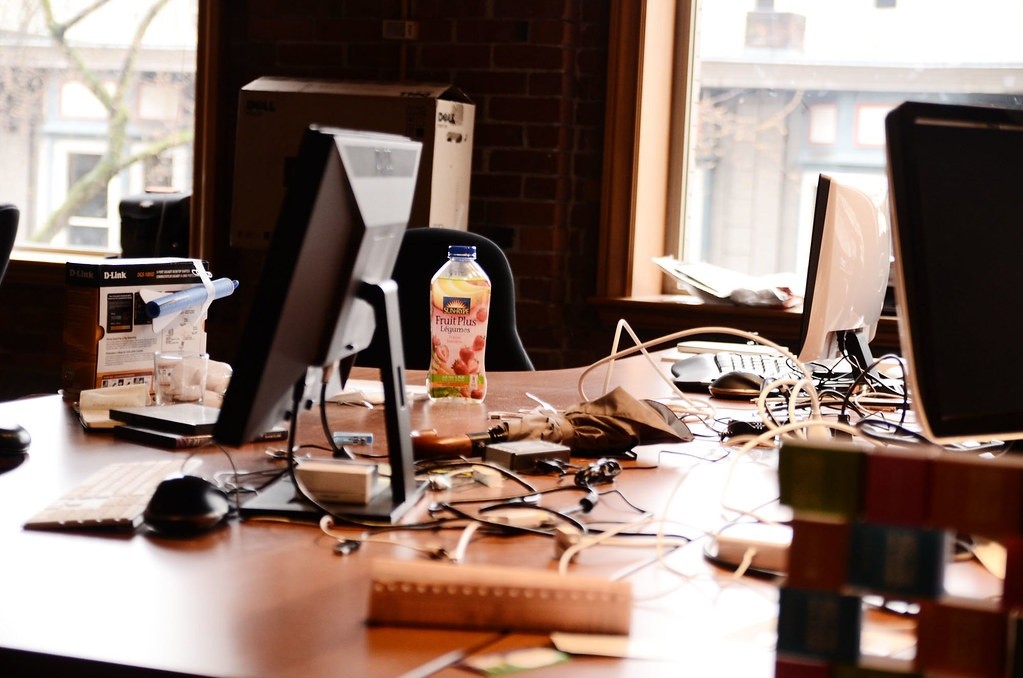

[212,126,430,524]
[886,99,1023,444]
[797,173,890,384]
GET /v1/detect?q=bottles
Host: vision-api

[428,245,492,403]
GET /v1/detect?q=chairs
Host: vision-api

[358,228,535,373]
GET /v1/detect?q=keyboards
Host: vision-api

[671,352,800,394]
[24,459,203,532]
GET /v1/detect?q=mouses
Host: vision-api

[709,372,764,399]
[144,476,231,533]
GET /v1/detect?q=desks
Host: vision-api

[0,367,1023,678]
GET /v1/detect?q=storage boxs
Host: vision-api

[226,75,476,247]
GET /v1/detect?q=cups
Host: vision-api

[151,353,212,407]
